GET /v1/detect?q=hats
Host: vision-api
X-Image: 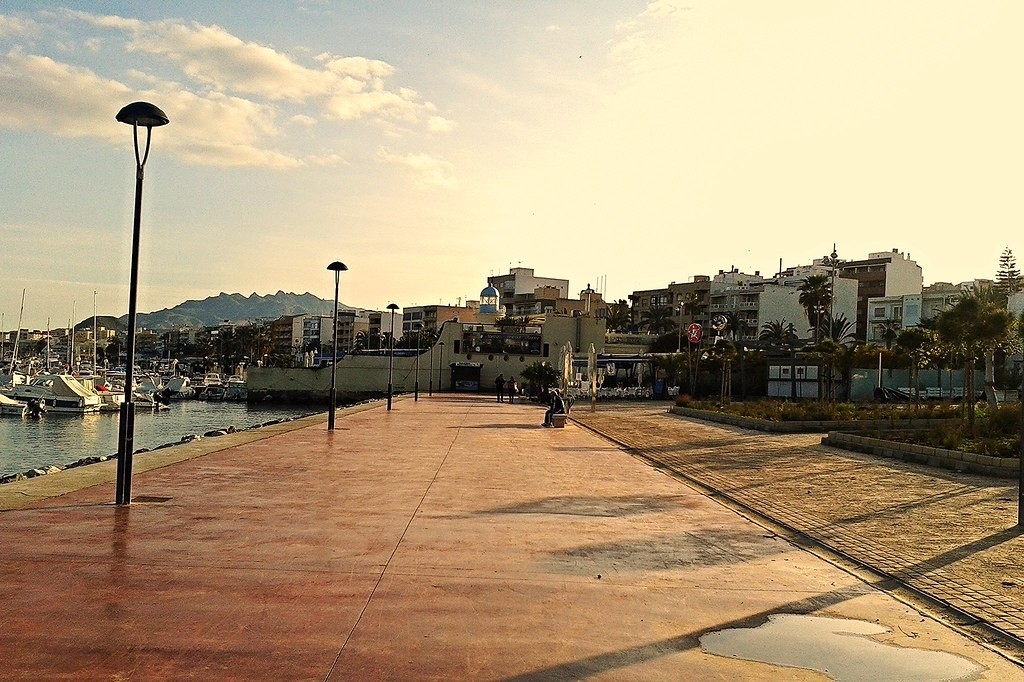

[509,376,513,378]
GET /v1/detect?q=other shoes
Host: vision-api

[501,401,504,402]
[542,422,550,426]
[497,401,499,402]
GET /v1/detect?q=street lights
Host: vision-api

[427,334,437,396]
[386,303,400,411]
[414,323,424,402]
[438,342,445,393]
[115,101,170,505]
[327,261,349,432]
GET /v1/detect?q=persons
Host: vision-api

[541,388,566,428]
[506,376,518,404]
[494,374,507,403]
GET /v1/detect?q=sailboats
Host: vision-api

[0,288,247,419]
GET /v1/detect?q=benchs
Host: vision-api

[900,388,926,399]
[552,413,567,428]
[927,388,951,399]
[954,388,967,399]
